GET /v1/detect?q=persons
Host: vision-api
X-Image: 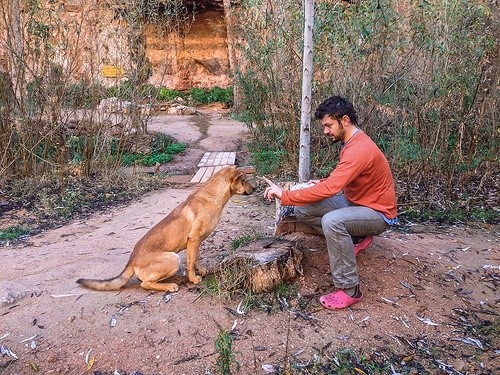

[262,96,397,308]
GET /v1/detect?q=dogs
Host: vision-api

[74,167,258,293]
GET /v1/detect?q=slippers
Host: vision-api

[320,289,363,309]
[352,236,373,256]
